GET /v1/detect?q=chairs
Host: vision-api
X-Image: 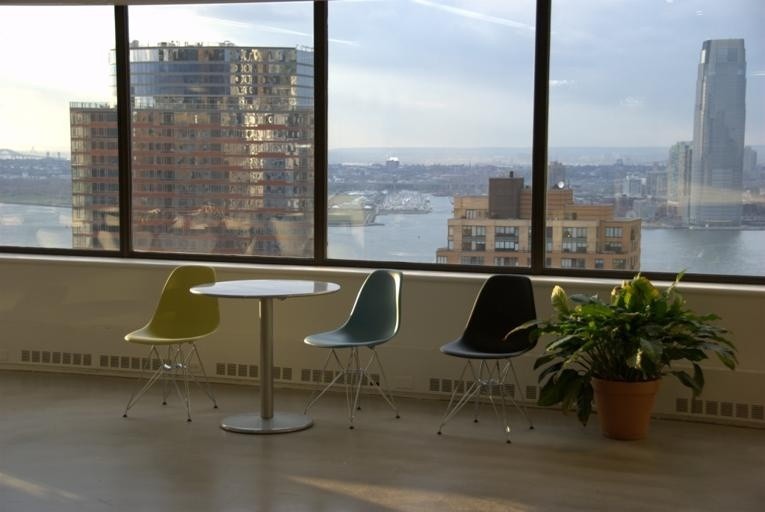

[304,265,407,429]
[437,273,539,444]
[121,265,222,423]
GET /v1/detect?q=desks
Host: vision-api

[188,278,346,438]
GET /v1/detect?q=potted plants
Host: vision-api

[502,271,745,441]
